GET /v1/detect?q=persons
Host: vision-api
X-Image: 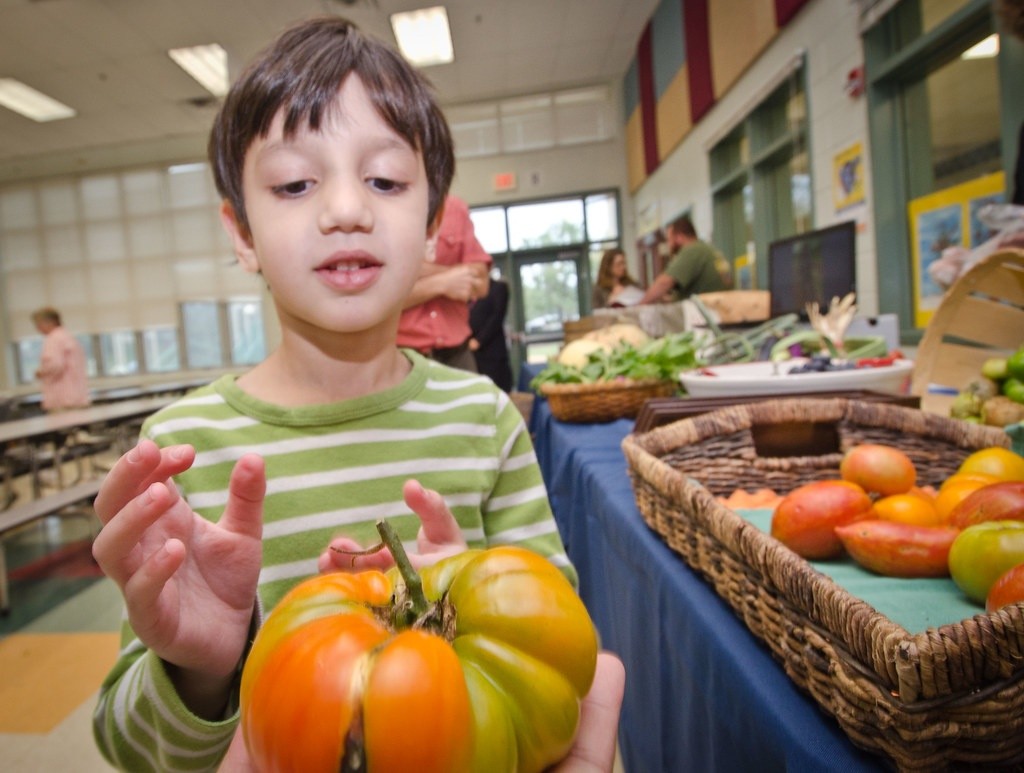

[468,275,515,394]
[592,248,648,310]
[29,308,92,412]
[396,195,494,372]
[640,219,729,306]
[91,13,626,773]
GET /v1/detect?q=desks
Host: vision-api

[518,362,885,773]
[0,377,216,449]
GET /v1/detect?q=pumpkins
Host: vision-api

[559,322,649,369]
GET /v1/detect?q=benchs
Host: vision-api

[0,441,111,483]
[0,472,106,537]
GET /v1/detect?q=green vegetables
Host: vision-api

[528,329,698,400]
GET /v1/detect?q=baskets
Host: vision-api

[621,397,1024,773]
[539,371,675,422]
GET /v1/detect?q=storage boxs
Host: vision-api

[697,290,770,325]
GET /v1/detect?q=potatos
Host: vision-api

[948,377,1024,431]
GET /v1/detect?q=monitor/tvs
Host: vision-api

[768,220,856,321]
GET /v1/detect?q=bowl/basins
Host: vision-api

[678,360,915,397]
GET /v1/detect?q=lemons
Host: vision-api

[980,342,1024,404]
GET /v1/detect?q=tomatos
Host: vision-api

[772,443,1024,618]
[239,543,599,773]
